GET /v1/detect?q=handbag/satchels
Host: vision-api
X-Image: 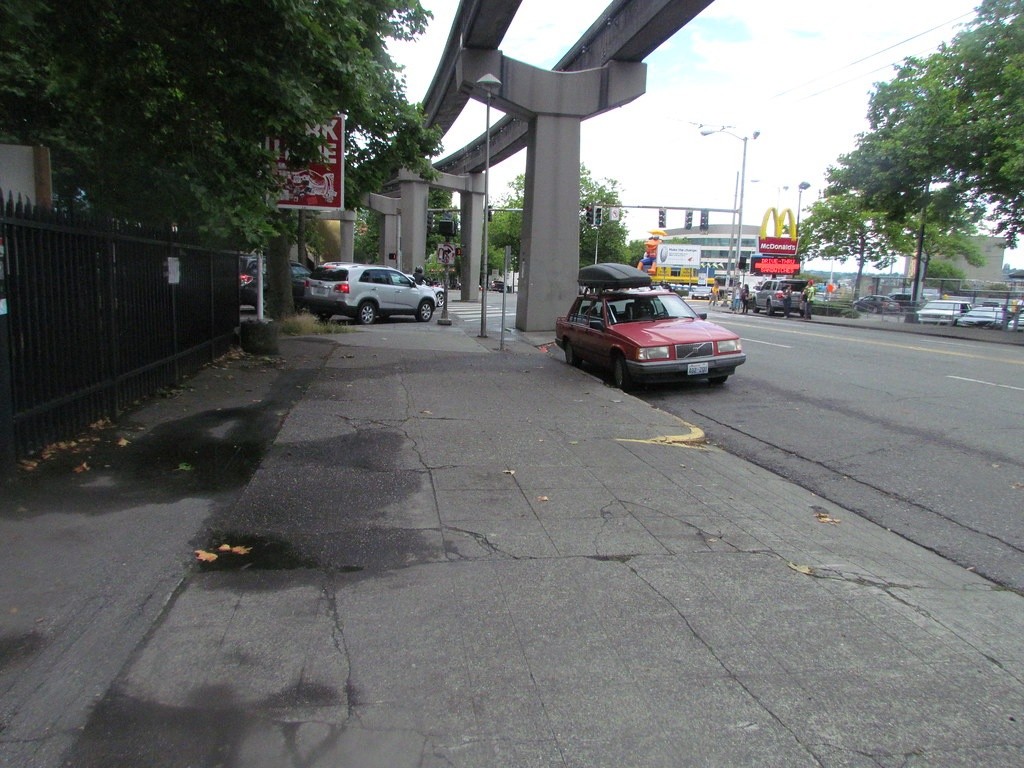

[729,300,733,309]
[740,300,743,309]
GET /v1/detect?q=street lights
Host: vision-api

[699,131,748,306]
[476,71,503,336]
[750,180,780,214]
[797,182,811,234]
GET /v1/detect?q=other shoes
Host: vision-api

[804,316,811,320]
[784,316,787,318]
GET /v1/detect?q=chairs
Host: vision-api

[624,303,640,320]
[600,305,617,323]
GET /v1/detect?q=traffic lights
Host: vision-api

[659,207,667,227]
[586,205,593,224]
[389,253,396,260]
[685,210,693,229]
[740,257,746,269]
[455,247,461,255]
[701,210,708,230]
[427,212,433,233]
[594,206,602,226]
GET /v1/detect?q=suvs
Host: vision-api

[303,262,439,325]
[239,254,313,314]
[888,294,928,311]
[751,279,809,316]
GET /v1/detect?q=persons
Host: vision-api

[413,265,433,285]
[731,282,750,314]
[799,280,834,320]
[708,281,719,306]
[781,284,792,318]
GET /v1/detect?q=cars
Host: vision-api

[691,286,712,300]
[393,274,444,308]
[554,262,746,391]
[955,302,1024,331]
[916,299,973,326]
[853,295,900,315]
[433,282,442,288]
[488,280,504,291]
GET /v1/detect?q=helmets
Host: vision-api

[415,266,423,272]
